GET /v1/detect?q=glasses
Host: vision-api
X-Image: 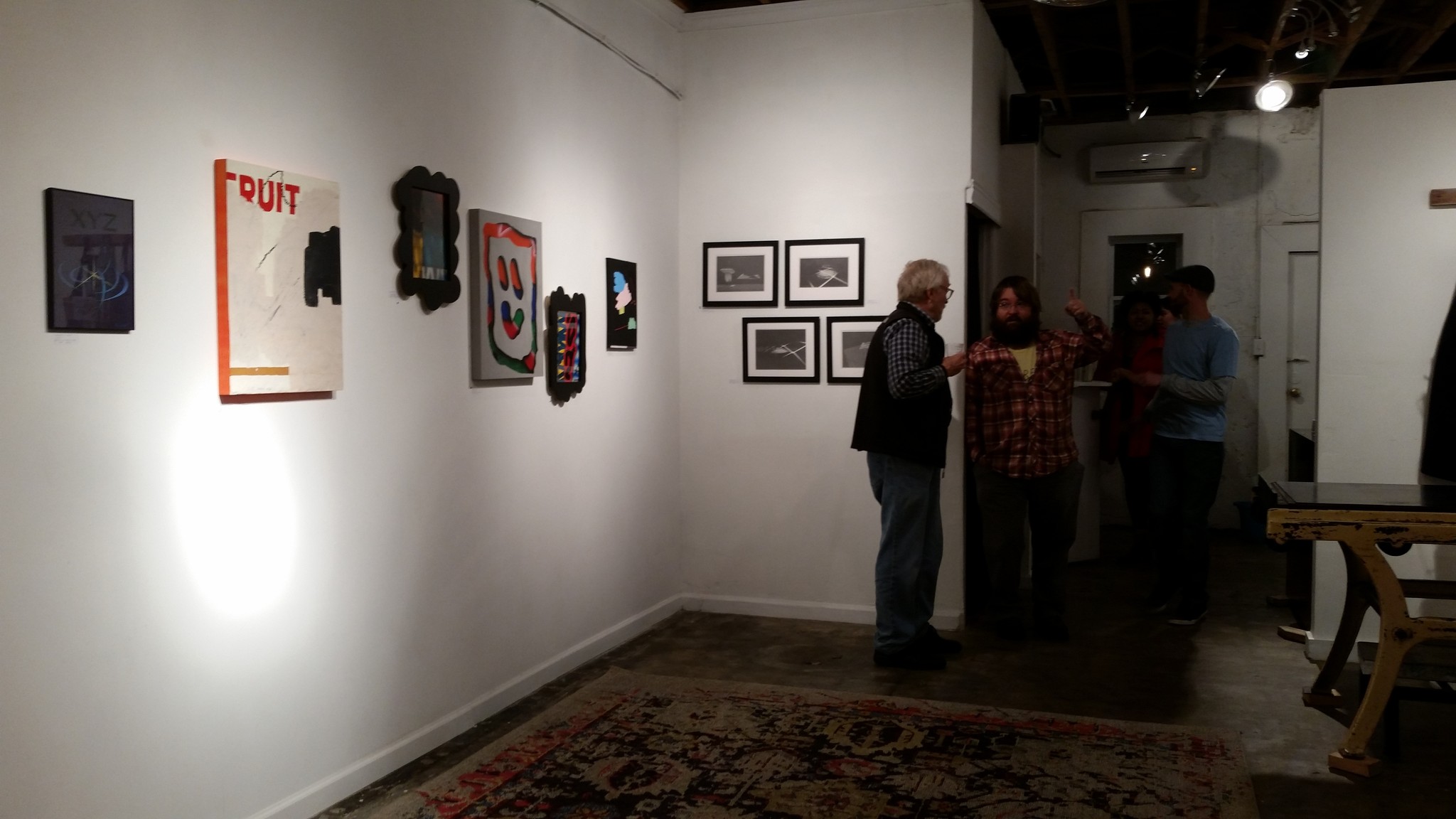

[939,285,953,300]
[995,301,1029,310]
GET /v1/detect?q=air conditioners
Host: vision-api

[1089,141,1206,184]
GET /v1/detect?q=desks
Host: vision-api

[1241,478,1456,776]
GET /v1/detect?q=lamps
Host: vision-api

[1189,64,1228,98]
[1126,96,1150,120]
[1288,0,1363,59]
[1251,71,1295,113]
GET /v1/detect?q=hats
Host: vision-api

[1169,264,1217,292]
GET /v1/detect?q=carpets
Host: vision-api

[361,667,1263,819]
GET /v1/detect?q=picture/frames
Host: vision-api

[784,237,865,307]
[742,316,821,383]
[826,315,889,383]
[701,240,778,306]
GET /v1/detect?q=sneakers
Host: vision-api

[875,624,966,670]
[1139,593,1210,626]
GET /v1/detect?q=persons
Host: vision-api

[1093,286,1162,567]
[1141,263,1238,625]
[847,257,970,674]
[970,273,1115,652]
[1128,294,1184,552]
[1408,282,1454,617]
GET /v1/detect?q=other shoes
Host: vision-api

[1002,614,1069,641]
